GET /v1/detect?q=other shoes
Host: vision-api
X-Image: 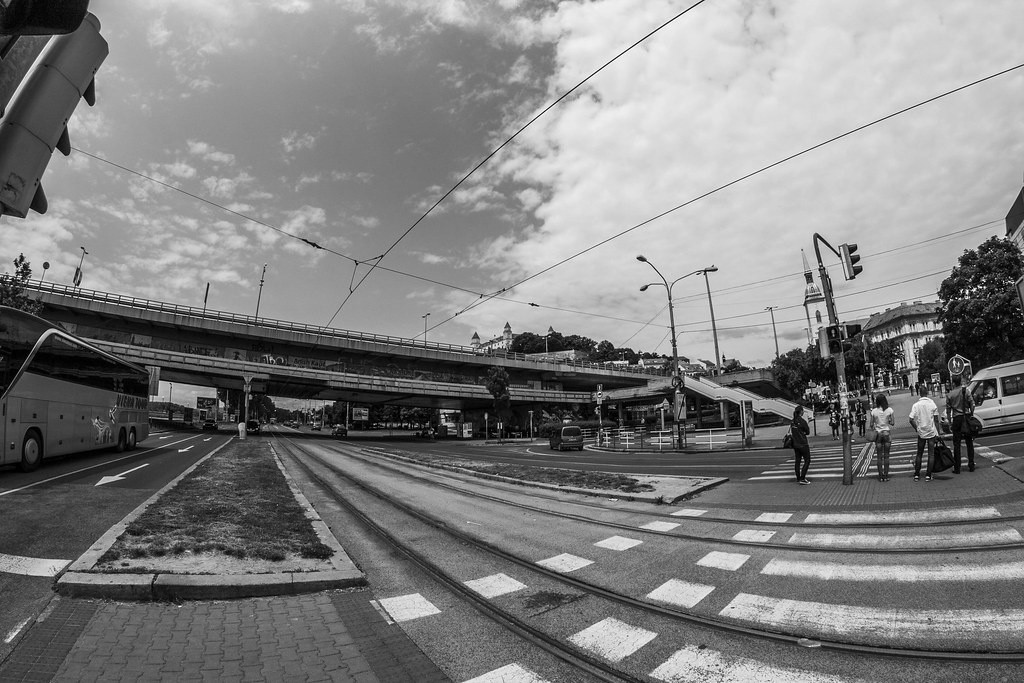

[913,476,918,481]
[879,478,889,482]
[951,470,960,473]
[925,475,934,482]
[796,476,811,485]
[969,467,976,471]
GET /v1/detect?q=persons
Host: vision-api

[790,406,811,484]
[870,394,895,481]
[979,382,996,401]
[909,378,949,398]
[829,399,866,440]
[909,387,943,481]
[947,375,977,474]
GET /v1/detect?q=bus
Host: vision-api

[0,304,150,471]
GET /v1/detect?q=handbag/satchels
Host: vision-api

[781,426,793,449]
[828,417,836,426]
[933,437,954,473]
[865,427,877,441]
[966,412,985,435]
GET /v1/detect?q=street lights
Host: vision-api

[636,255,720,446]
[422,312,431,347]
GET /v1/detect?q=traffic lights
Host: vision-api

[838,243,863,281]
[844,323,862,339]
[0,9,109,218]
[826,325,842,353]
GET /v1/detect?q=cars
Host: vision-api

[331,424,348,436]
[941,359,1024,433]
[549,426,583,451]
